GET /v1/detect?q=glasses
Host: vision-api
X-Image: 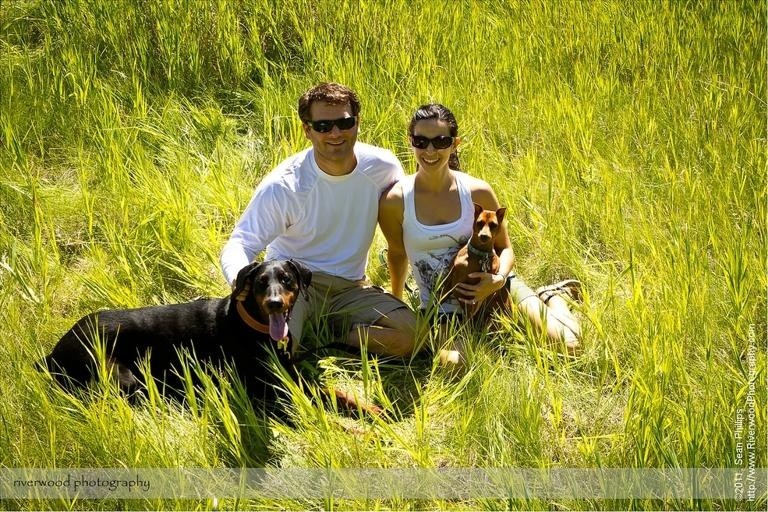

[305,117,356,132]
[411,135,453,150]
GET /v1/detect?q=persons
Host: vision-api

[368,101,585,376]
[216,81,431,365]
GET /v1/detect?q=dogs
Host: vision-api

[32,260,395,448]
[435,203,512,320]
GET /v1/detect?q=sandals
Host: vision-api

[535,279,581,303]
